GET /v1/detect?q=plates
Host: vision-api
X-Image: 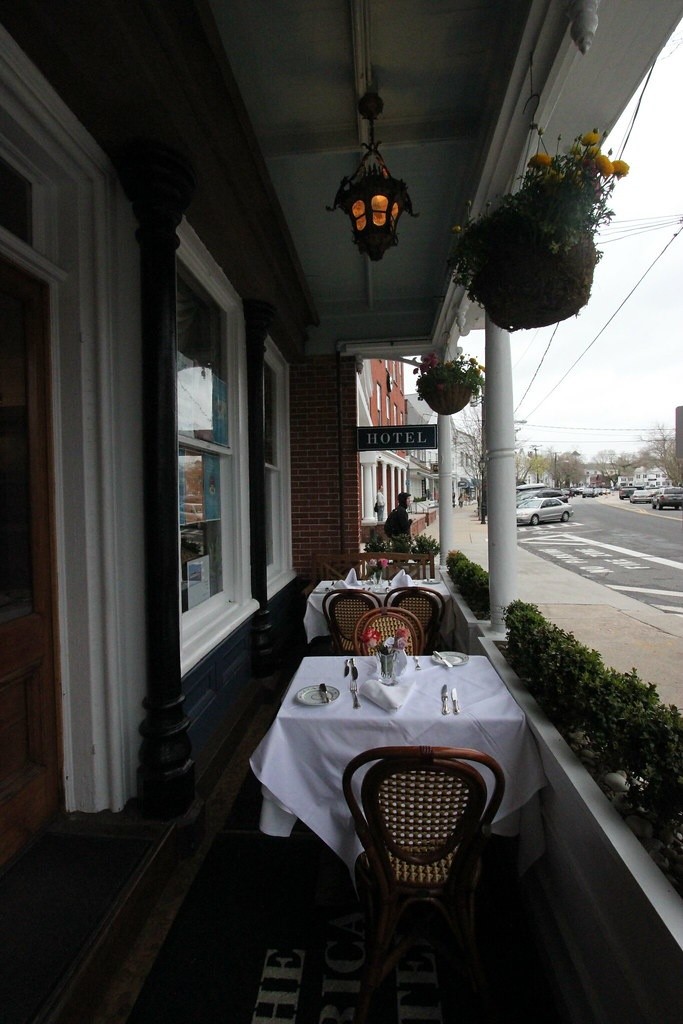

[373,587,392,594]
[313,587,334,593]
[296,685,340,705]
[432,652,470,666]
[422,579,440,584]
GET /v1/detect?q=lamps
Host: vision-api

[325,92,421,264]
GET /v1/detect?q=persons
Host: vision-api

[452,489,455,507]
[458,493,463,506]
[678,482,683,488]
[376,486,385,521]
[393,492,416,565]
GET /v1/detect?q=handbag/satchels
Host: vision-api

[374,503,379,512]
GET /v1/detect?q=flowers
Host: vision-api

[370,558,388,584]
[360,625,410,678]
[410,349,487,400]
[445,119,628,306]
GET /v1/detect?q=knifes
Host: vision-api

[350,658,358,680]
[345,660,350,676]
[433,651,452,667]
[320,683,331,703]
[442,685,450,715]
[451,688,460,714]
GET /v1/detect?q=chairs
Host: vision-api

[300,554,368,596]
[368,550,436,579]
[343,745,505,1024]
[384,587,446,655]
[323,589,382,656]
[354,606,425,658]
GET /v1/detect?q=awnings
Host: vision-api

[458,477,481,489]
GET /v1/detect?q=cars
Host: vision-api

[515,481,610,506]
[629,490,652,503]
[515,498,574,526]
[618,486,644,500]
[180,503,204,528]
[651,487,683,510]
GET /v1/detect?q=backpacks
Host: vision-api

[384,508,407,539]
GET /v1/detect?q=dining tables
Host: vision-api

[248,655,549,900]
[302,578,454,644]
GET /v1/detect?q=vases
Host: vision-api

[471,237,602,333]
[425,385,471,415]
[376,653,396,685]
[371,571,383,585]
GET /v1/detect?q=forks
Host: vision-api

[415,660,421,671]
[350,680,361,709]
